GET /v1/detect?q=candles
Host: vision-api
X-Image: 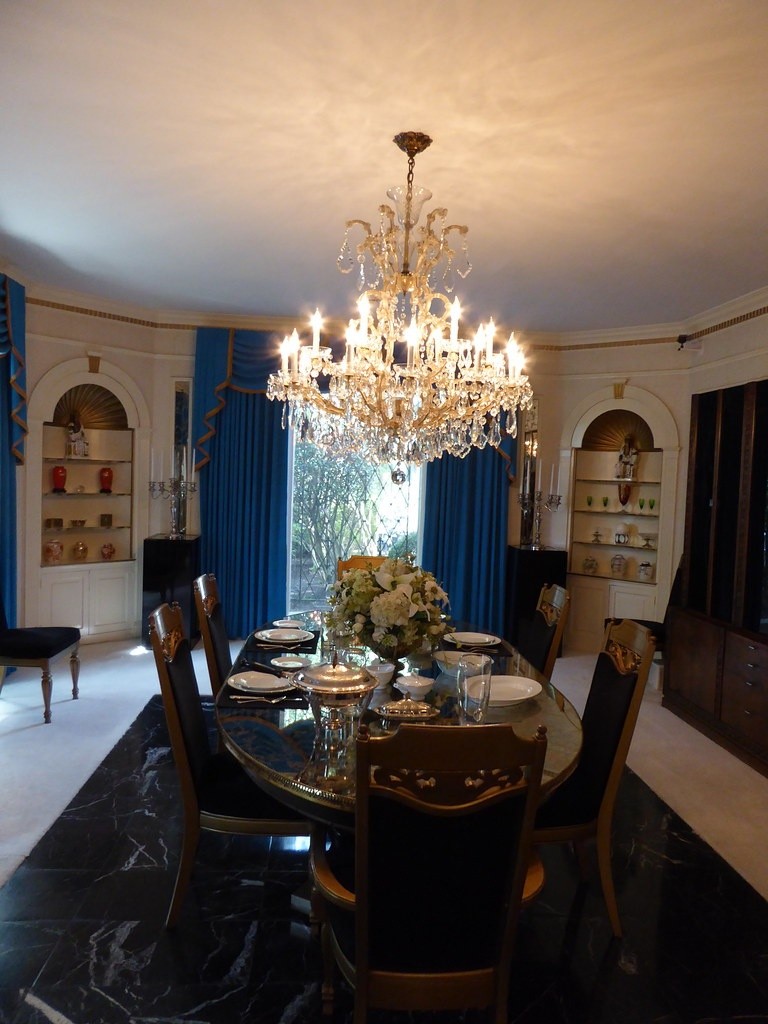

[557,464,560,495]
[151,448,154,481]
[183,446,188,481]
[161,449,163,482]
[550,464,554,494]
[538,459,541,490]
[520,465,523,493]
[526,461,529,493]
[192,448,195,482]
[171,446,175,477]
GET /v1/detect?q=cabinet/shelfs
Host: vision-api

[38,564,138,634]
[569,447,663,583]
[568,581,656,651]
[39,422,136,567]
[660,377,768,780]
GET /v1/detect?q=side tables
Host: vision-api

[142,533,202,651]
[506,543,569,661]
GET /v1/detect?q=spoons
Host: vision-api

[257,644,300,650]
[238,696,286,704]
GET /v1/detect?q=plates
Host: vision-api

[261,630,309,640]
[227,670,296,693]
[449,633,495,644]
[272,620,303,628]
[373,706,440,720]
[254,629,315,643]
[444,632,502,646]
[234,672,289,689]
[462,674,542,706]
[270,657,311,668]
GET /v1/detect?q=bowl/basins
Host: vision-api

[433,673,462,697]
[367,665,394,689]
[433,650,490,678]
[71,519,86,527]
[396,676,434,700]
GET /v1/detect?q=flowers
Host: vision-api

[328,557,458,647]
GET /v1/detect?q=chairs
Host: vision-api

[533,616,656,943]
[337,556,387,580]
[604,548,683,694]
[0,597,81,724]
[525,582,569,682]
[193,574,233,752]
[148,601,314,927]
[308,724,548,1024]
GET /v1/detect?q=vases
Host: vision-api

[359,635,423,669]
[51,466,67,493]
[45,539,63,563]
[71,541,88,561]
[583,558,598,574]
[610,554,625,577]
[101,543,115,560]
[638,561,652,580]
[100,468,112,493]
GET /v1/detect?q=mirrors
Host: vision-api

[172,378,192,479]
[517,394,542,500]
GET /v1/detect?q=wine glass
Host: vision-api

[602,497,608,511]
[587,495,592,510]
[638,498,644,514]
[648,499,655,514]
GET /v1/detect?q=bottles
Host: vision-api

[583,556,597,574]
[611,555,626,575]
[101,543,116,560]
[639,561,653,580]
[44,539,63,564]
[52,465,66,488]
[73,542,88,560]
[100,467,113,489]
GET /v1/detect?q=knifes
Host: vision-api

[229,695,303,701]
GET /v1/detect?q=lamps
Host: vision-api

[265,131,534,464]
[676,333,706,359]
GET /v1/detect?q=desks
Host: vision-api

[211,610,585,829]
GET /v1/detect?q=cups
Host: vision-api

[456,653,491,726]
